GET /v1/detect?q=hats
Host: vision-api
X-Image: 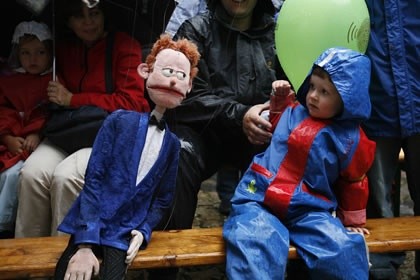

[12,20,52,45]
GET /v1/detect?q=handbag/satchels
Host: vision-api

[44,103,110,155]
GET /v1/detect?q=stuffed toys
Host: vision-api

[53,33,201,280]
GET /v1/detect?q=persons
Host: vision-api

[0,19,57,238]
[364,0,420,280]
[223,47,379,280]
[16,0,147,239]
[164,0,285,231]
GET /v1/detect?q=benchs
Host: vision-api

[0,215,420,280]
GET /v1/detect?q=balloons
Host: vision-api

[275,0,372,94]
